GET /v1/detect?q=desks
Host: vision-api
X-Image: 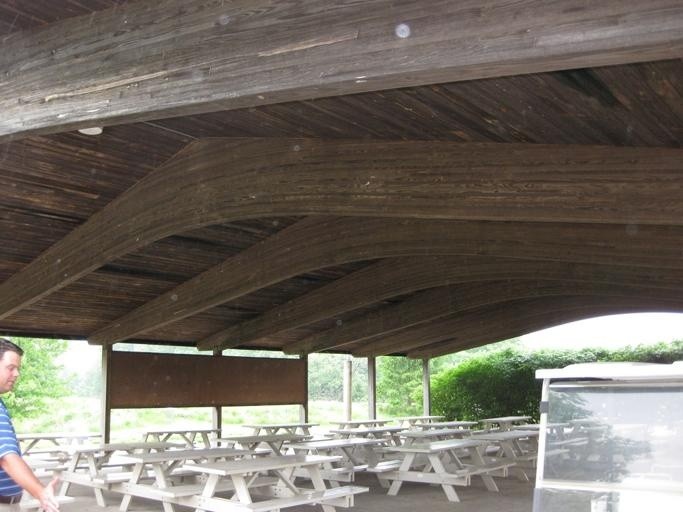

[13,410,370,512]
[361,414,606,502]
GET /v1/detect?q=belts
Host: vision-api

[0,493,24,505]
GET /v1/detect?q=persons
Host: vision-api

[0,339,63,511]
